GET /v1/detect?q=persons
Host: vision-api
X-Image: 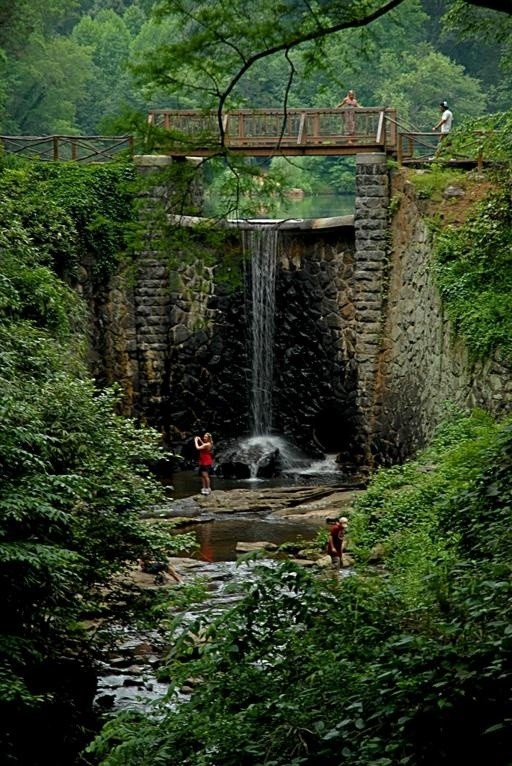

[336,89,363,144]
[194,432,214,495]
[428,102,457,162]
[327,517,348,569]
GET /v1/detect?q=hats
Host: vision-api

[338,517,349,528]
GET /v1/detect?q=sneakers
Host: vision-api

[201,487,211,495]
